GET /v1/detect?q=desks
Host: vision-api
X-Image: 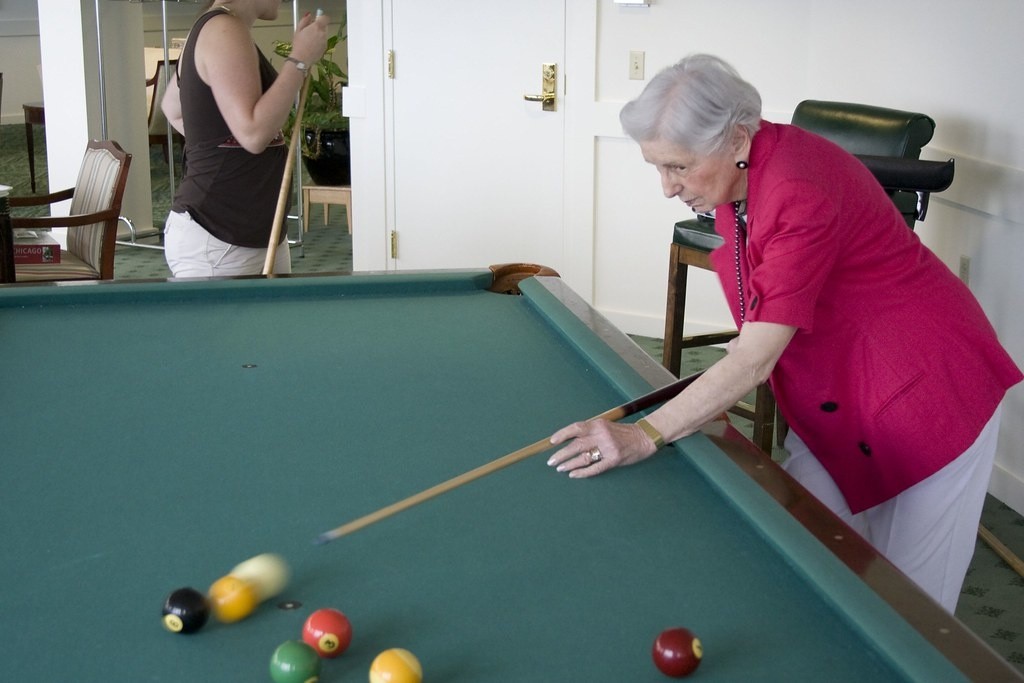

[22,103,45,193]
[302,178,352,235]
[0,264,1024,683]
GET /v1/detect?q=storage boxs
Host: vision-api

[13,230,60,264]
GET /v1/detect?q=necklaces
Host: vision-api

[218,4,236,17]
[732,201,748,323]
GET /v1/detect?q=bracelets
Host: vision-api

[636,419,666,451]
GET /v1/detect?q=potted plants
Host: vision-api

[266,6,352,188]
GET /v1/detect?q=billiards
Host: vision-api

[269,638,322,683]
[651,627,704,677]
[161,587,210,634]
[302,607,353,659]
[369,647,424,683]
[207,553,288,625]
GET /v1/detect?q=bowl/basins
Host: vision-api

[0,185,13,197]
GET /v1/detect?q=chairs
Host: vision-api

[663,98,955,456]
[145,60,187,177]
[0,139,132,285]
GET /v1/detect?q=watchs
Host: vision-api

[285,57,309,78]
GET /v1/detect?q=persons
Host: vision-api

[543,51,1024,616]
[162,0,330,279]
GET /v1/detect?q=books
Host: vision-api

[14,231,61,263]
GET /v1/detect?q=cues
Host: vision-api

[260,7,325,275]
[320,366,713,543]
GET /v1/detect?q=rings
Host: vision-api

[585,447,602,462]
